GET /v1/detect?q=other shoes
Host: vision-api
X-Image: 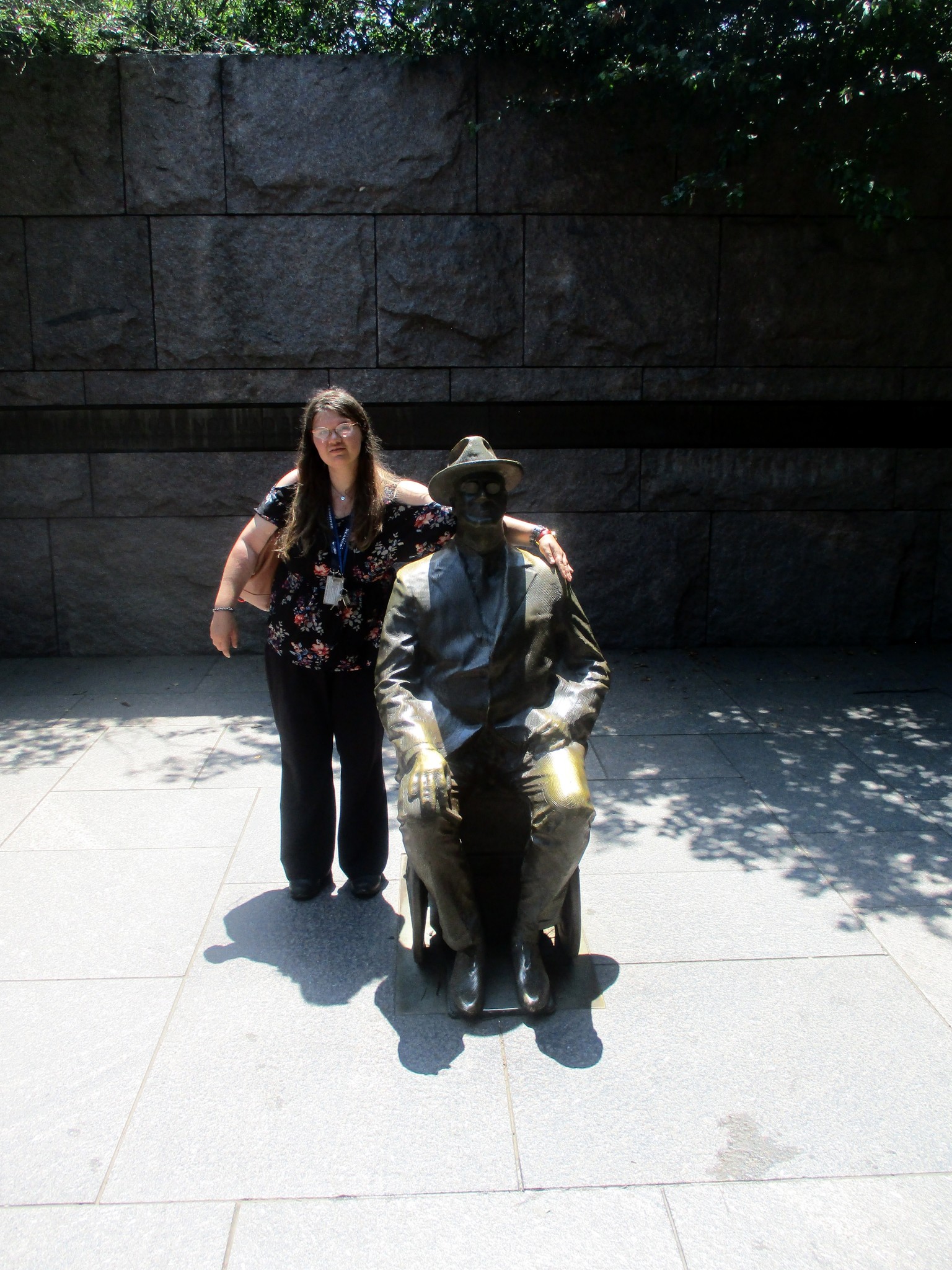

[288,868,331,901]
[350,875,382,896]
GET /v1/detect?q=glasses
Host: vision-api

[311,423,357,440]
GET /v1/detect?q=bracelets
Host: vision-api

[211,608,234,614]
[530,525,546,544]
[537,529,561,545]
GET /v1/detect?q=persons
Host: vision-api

[210,386,575,902]
[373,435,611,1023]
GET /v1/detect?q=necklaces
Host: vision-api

[330,482,354,502]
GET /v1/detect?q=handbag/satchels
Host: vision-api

[239,516,294,612]
